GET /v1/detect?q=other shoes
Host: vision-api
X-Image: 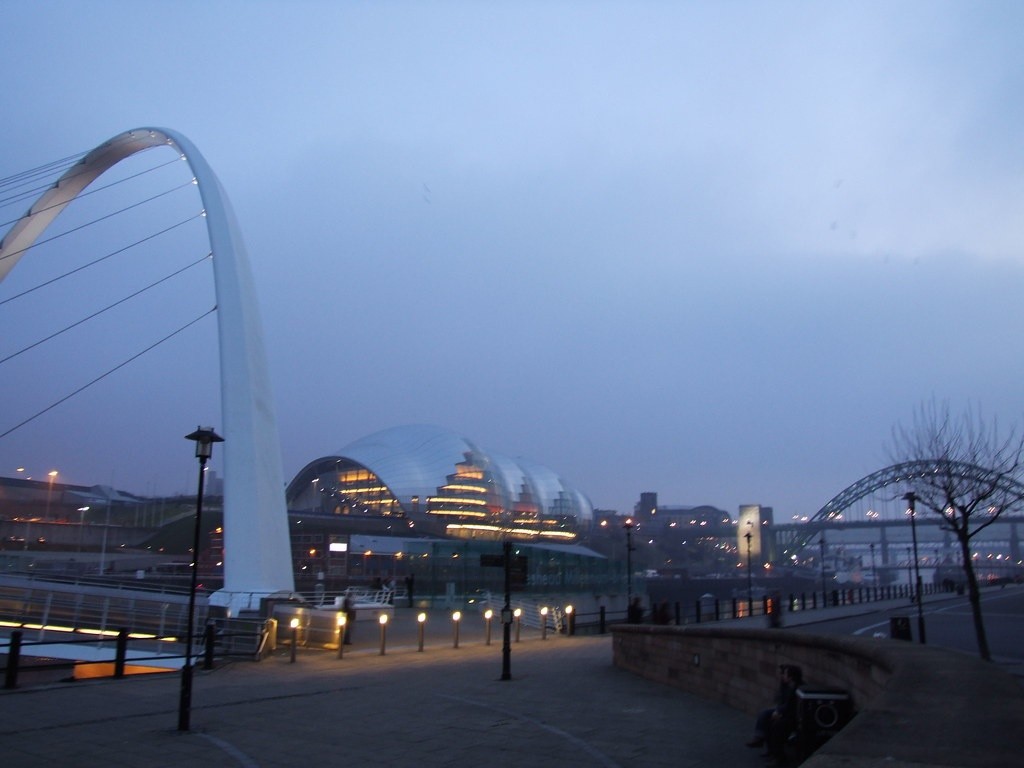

[746,738,764,747]
[408,605,412,608]
[345,642,353,646]
[765,761,783,767]
[760,752,770,757]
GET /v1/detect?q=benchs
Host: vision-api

[750,704,796,746]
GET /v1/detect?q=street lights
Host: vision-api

[743,520,755,617]
[178,425,227,731]
[901,492,926,645]
[624,519,635,624]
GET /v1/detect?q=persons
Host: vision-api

[627,597,644,624]
[404,573,415,608]
[656,603,671,624]
[342,592,355,645]
[370,577,382,589]
[745,664,802,758]
[769,598,782,628]
[943,577,954,592]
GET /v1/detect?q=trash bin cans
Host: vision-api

[794,685,852,763]
[888,615,913,641]
